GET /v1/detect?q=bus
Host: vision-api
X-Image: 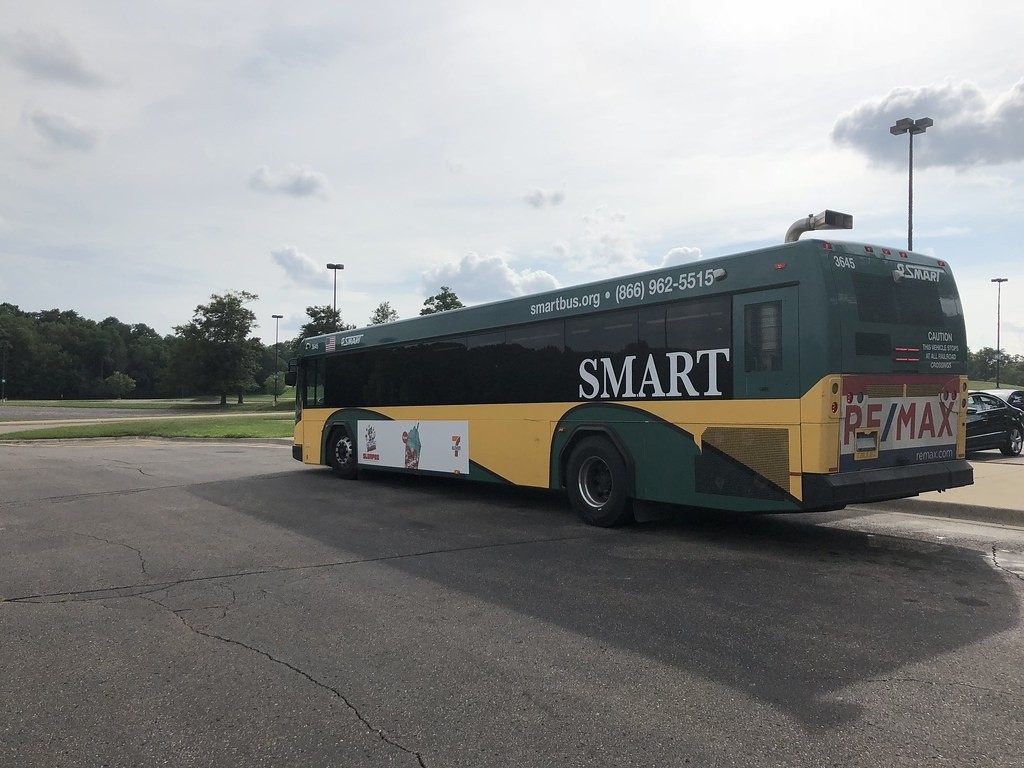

[283,238,975,528]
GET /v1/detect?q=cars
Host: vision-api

[978,389,1024,411]
[964,390,1024,457]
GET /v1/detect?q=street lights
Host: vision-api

[991,278,1008,389]
[326,263,344,334]
[272,315,284,407]
[890,117,933,251]
